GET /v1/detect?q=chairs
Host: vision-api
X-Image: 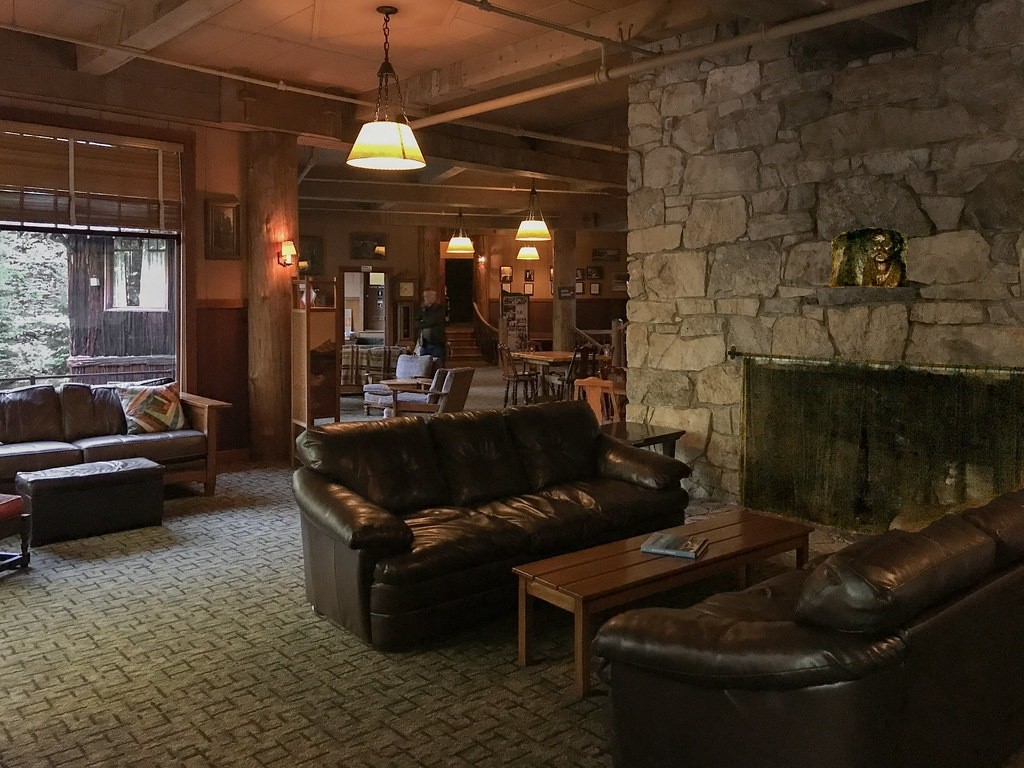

[360,353,438,415]
[573,377,621,426]
[497,343,540,409]
[382,366,475,418]
[0,493,32,574]
[548,344,597,403]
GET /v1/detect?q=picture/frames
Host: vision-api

[590,283,601,295]
[575,268,585,281]
[607,271,629,292]
[525,270,534,281]
[524,283,534,296]
[587,266,604,280]
[550,268,554,281]
[204,198,247,261]
[501,283,511,293]
[500,266,513,282]
[298,235,327,276]
[557,286,576,299]
[349,231,386,260]
[551,283,554,295]
[576,282,584,294]
[592,249,620,262]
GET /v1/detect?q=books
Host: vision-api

[641,533,709,558]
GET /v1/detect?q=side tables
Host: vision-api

[604,422,687,460]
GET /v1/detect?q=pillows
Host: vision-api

[396,353,434,379]
[426,368,452,404]
[115,381,189,435]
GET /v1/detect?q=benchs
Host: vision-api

[13,457,164,549]
[0,376,233,498]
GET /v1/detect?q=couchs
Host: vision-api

[589,487,1024,768]
[289,399,693,650]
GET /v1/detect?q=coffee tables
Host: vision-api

[510,510,818,699]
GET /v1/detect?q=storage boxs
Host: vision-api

[392,277,419,302]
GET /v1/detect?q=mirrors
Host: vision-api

[336,264,393,399]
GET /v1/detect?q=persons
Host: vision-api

[415,287,446,376]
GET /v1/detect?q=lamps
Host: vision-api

[445,207,476,254]
[516,241,540,260]
[344,5,426,172]
[514,178,552,242]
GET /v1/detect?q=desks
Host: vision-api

[582,386,627,419]
[510,350,600,397]
